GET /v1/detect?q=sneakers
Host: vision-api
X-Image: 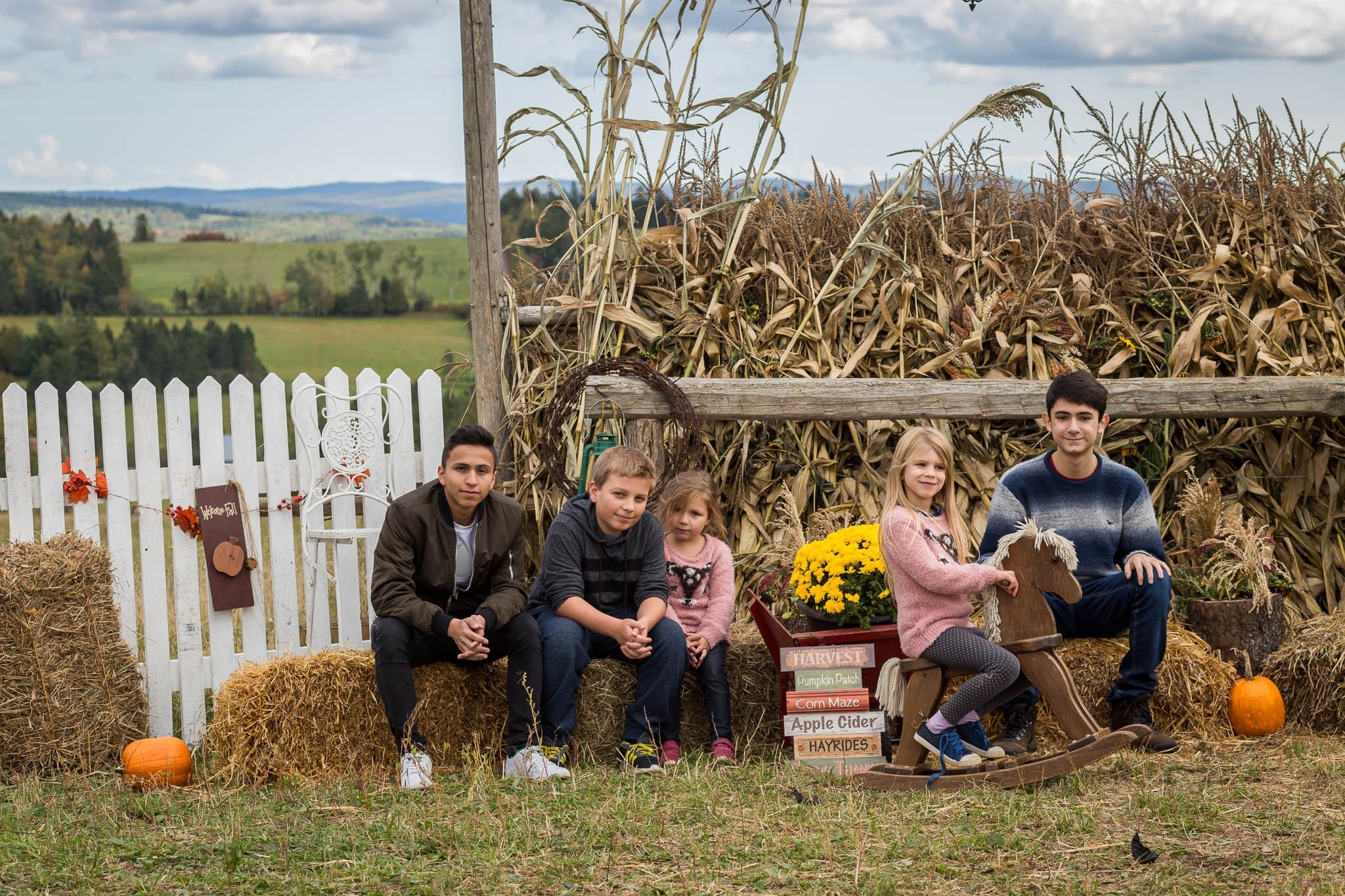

[661,741,680,767]
[541,744,569,768]
[616,740,661,775]
[505,745,570,780]
[1109,696,1178,756]
[712,737,738,765]
[915,722,983,790]
[398,754,432,792]
[990,707,1038,756]
[954,719,1006,758]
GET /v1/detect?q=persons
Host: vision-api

[654,470,737,766]
[878,426,1033,771]
[371,424,571,789]
[975,369,1178,755]
[523,446,689,774]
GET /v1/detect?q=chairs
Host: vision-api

[291,383,407,656]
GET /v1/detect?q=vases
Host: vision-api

[798,593,904,632]
[1184,586,1293,672]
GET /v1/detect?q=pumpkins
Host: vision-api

[1227,650,1286,737]
[114,736,194,791]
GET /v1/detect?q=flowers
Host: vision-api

[793,524,914,609]
[1170,479,1298,619]
